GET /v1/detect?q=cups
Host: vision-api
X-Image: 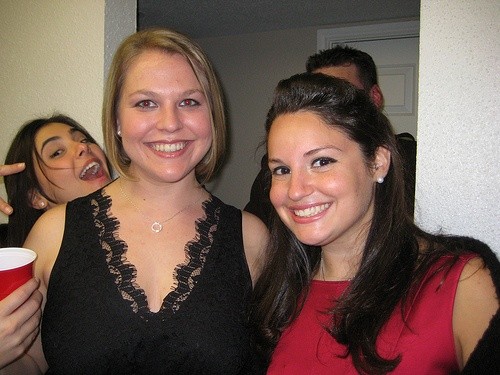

[0,247,38,301]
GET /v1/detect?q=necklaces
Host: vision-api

[119,186,206,233]
[320,262,357,314]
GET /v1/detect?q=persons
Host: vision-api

[0,27,290,375]
[242,71,500,375]
[0,111,115,248]
[245,43,418,222]
